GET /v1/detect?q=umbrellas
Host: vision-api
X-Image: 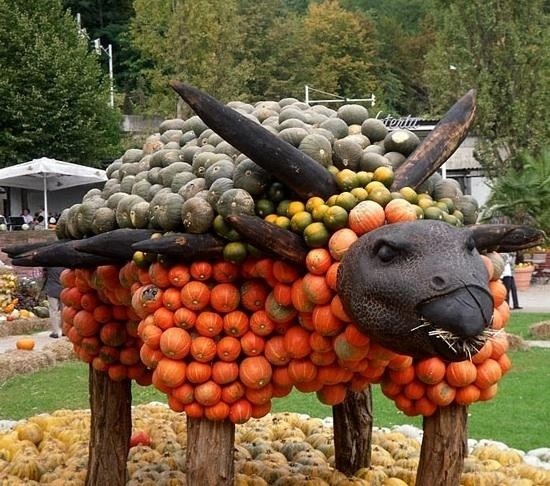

[0,157,108,229]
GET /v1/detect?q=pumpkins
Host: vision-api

[1,401,550,486]
[56,97,513,422]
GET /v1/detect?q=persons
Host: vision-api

[499,252,522,309]
[13,209,62,232]
